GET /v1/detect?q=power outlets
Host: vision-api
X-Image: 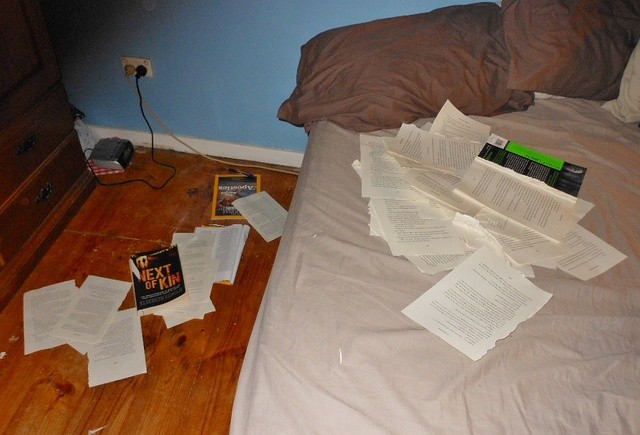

[119,56,153,79]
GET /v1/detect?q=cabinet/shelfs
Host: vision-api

[0,0,98,315]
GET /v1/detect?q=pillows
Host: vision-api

[276,2,535,133]
[500,1,640,102]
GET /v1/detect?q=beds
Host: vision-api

[229,32,640,435]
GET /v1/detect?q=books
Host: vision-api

[451,132,590,243]
[192,223,251,286]
[128,243,190,317]
[211,173,261,220]
